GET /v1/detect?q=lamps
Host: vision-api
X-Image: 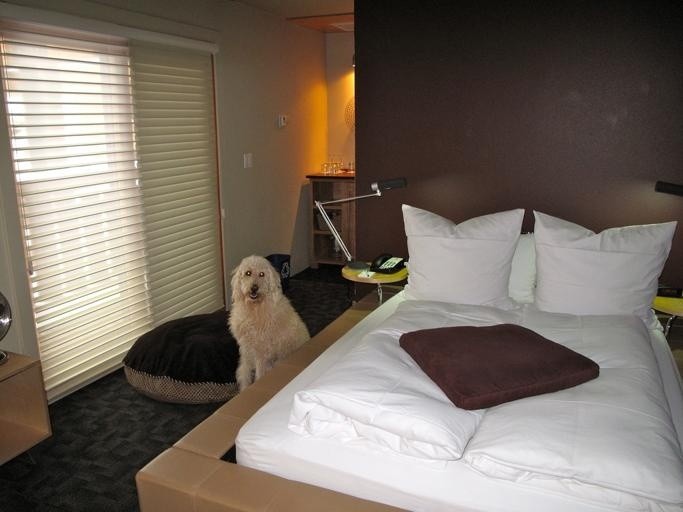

[314,177,405,269]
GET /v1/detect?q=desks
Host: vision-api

[341,262,408,310]
[653,297,682,339]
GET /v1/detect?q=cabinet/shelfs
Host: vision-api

[306,172,355,269]
[0,71,54,469]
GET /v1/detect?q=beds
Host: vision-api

[138,283,682,512]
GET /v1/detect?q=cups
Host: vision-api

[321,161,355,174]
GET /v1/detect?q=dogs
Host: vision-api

[227,254,311,394]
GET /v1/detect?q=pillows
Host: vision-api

[400,204,678,316]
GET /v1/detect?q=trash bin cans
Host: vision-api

[264,254,290,294]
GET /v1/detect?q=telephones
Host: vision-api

[370,253,406,274]
